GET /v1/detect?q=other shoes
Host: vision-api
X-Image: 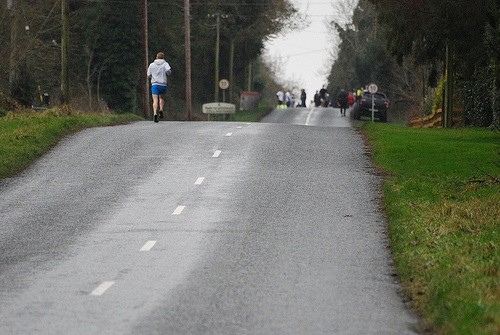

[159,111,163,119]
[155,115,158,123]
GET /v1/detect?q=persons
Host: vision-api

[147,52,172,123]
[314,85,330,107]
[338,86,363,116]
[276,88,306,108]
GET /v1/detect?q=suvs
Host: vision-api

[349,89,390,122]
